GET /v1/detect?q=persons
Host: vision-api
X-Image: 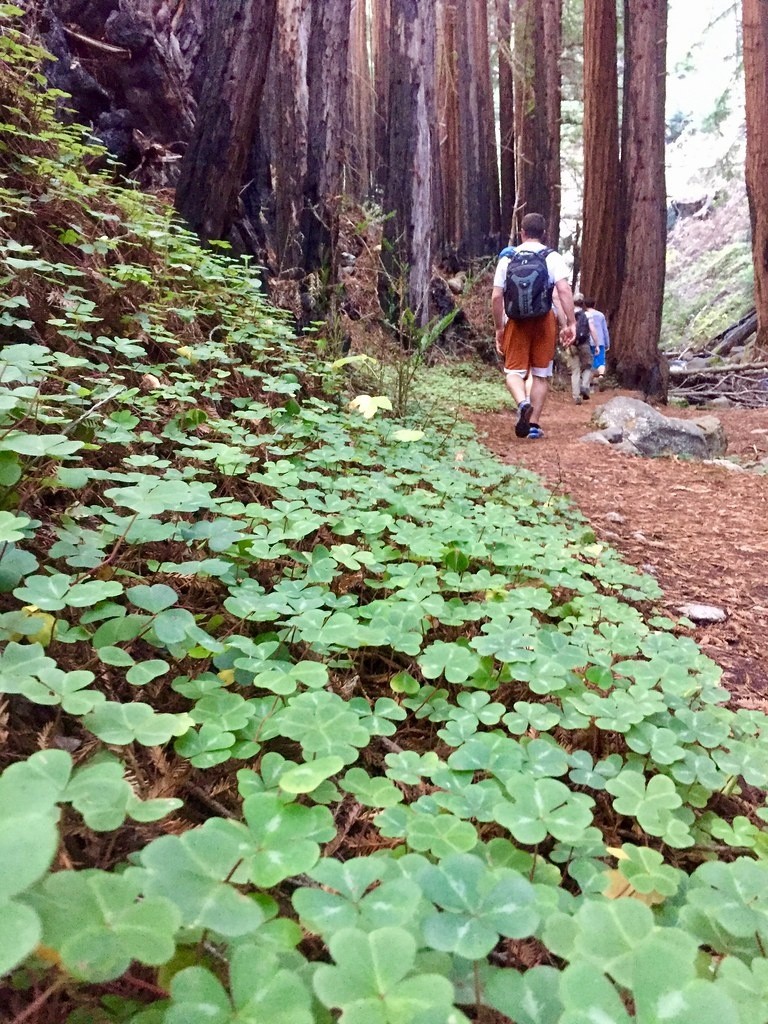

[500,249,570,420]
[578,297,611,386]
[560,292,600,408]
[490,212,579,440]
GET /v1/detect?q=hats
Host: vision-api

[573,292,583,302]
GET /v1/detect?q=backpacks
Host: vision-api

[498,246,555,319]
[567,311,590,346]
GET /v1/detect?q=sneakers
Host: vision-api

[580,386,590,399]
[514,398,533,438]
[527,424,545,438]
[597,374,605,392]
[575,398,582,405]
